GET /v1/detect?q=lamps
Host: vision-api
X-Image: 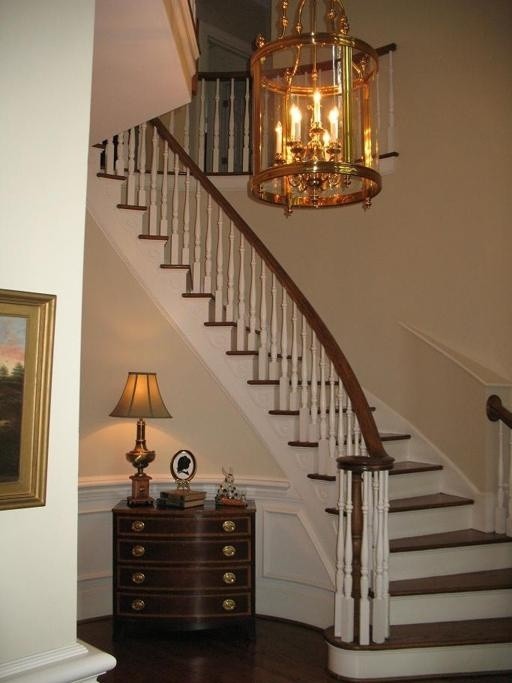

[242,0,384,218]
[107,369,174,505]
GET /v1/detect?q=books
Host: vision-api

[160,488,208,502]
[157,497,205,509]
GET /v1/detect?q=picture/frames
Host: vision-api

[0,287,59,510]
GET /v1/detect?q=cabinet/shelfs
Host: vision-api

[110,497,257,644]
